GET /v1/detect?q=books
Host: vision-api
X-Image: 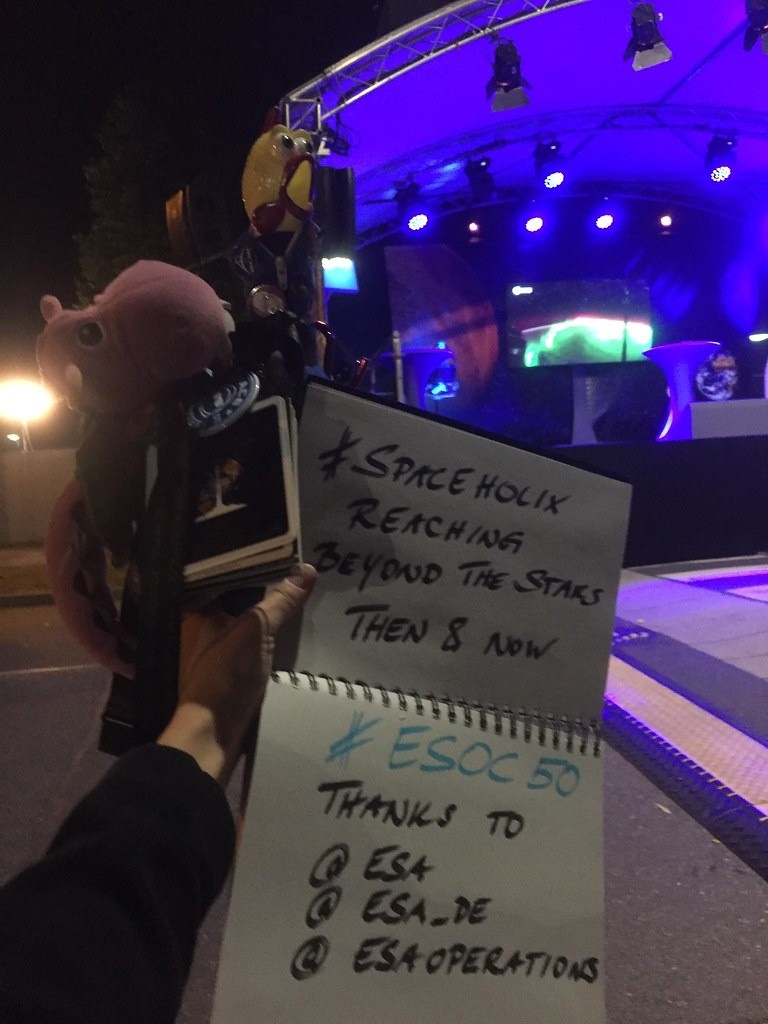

[208,377,633,1024]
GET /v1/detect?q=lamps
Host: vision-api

[623,3,674,71]
[705,130,737,183]
[485,32,532,113]
[532,136,567,191]
[399,198,434,234]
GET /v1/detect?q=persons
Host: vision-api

[0,562,320,1024]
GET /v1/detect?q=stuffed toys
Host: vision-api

[242,125,315,234]
[36,261,233,675]
